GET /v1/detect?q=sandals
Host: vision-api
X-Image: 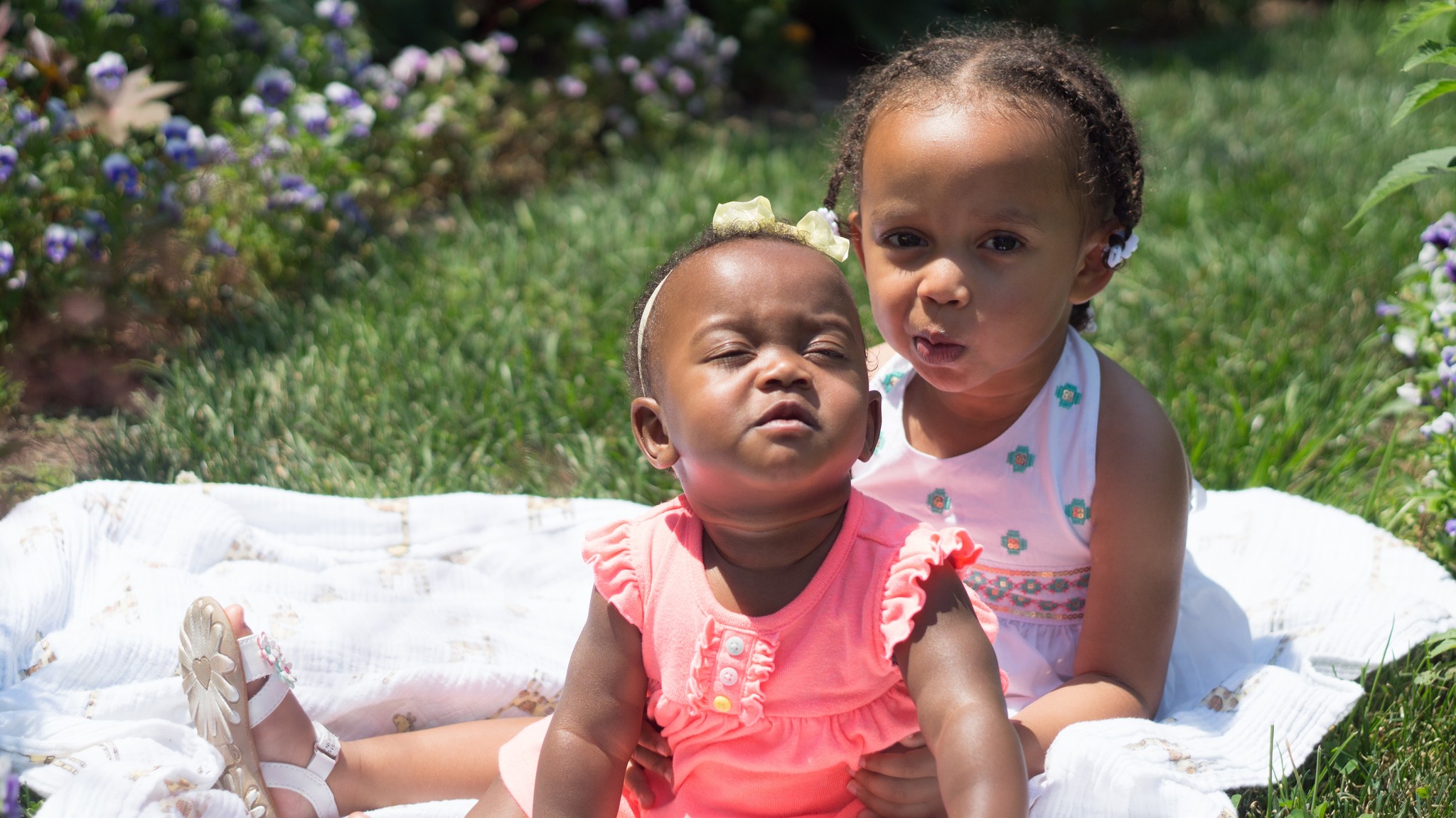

[179,595,341,816]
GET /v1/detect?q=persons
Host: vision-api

[176,26,1244,818]
[531,191,1032,818]
